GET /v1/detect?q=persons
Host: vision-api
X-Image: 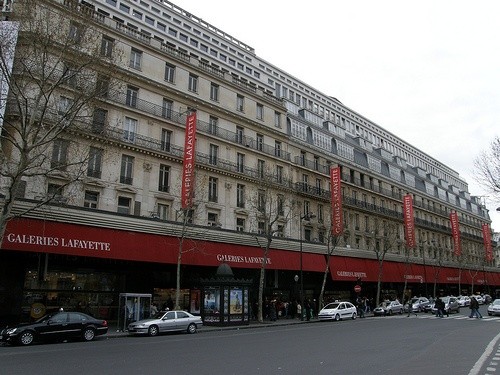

[379,288,473,307]
[490,288,500,301]
[468,297,482,319]
[434,297,449,318]
[58,286,170,320]
[482,291,492,305]
[249,294,376,321]
[406,303,417,317]
[473,287,489,297]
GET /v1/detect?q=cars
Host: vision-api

[458,294,491,307]
[4,312,109,346]
[432,297,460,313]
[127,311,203,336]
[404,297,429,313]
[374,301,404,314]
[487,299,500,316]
[318,302,357,320]
